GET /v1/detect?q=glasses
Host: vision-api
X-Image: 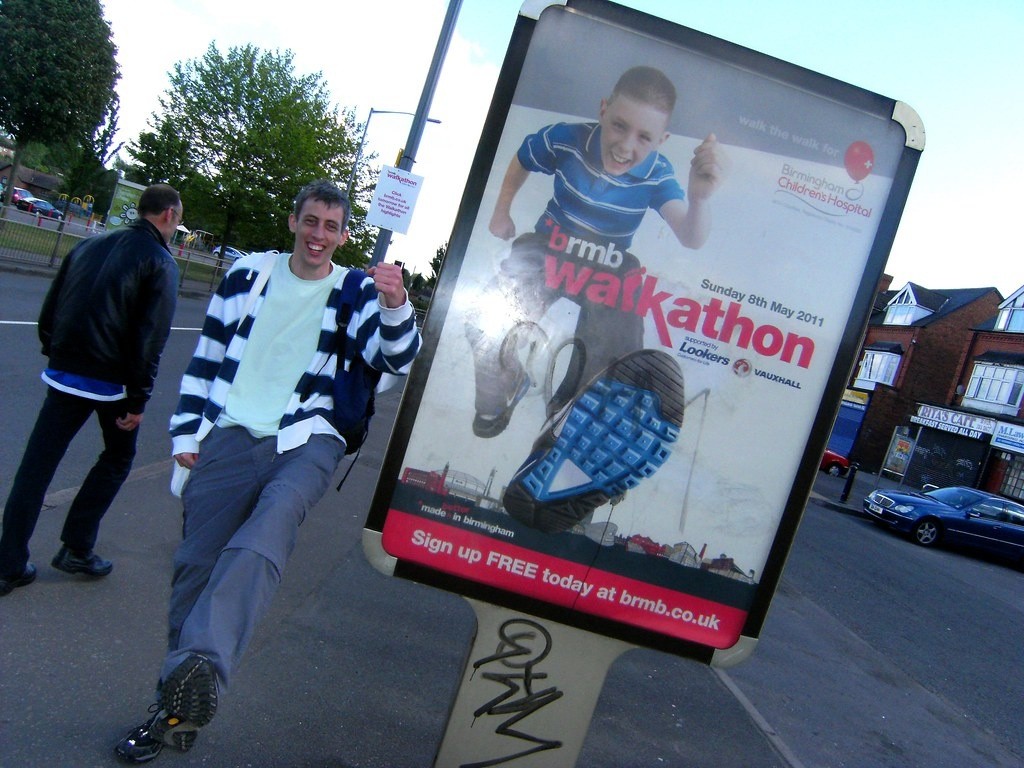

[166,207,184,225]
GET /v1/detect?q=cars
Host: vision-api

[1,186,35,205]
[817,449,849,478]
[212,245,250,260]
[17,197,64,220]
[863,485,1024,568]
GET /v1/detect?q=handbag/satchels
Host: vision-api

[334,265,381,452]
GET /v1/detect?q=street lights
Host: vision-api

[346,107,440,194]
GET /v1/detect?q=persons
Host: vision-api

[0,183,183,599]
[114,182,422,763]
[466,65,730,537]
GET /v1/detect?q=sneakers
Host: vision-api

[0,562,36,595]
[150,655,218,753]
[504,349,685,537]
[53,545,113,575]
[474,372,529,438]
[117,722,163,763]
